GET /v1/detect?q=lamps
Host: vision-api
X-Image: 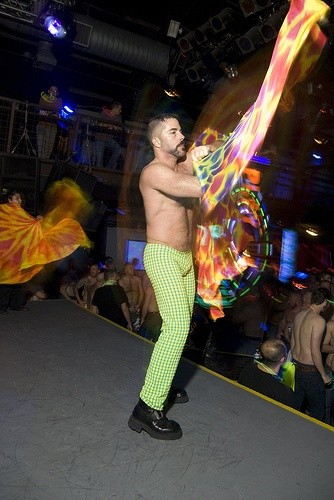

[44,9,77,47]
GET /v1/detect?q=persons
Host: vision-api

[59,257,163,342]
[184,269,334,427]
[96,101,122,169]
[1,190,47,314]
[36,86,62,159]
[127,114,226,440]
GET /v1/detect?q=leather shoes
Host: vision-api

[0,309,9,315]
[129,398,183,440]
[166,389,189,403]
[9,305,28,311]
